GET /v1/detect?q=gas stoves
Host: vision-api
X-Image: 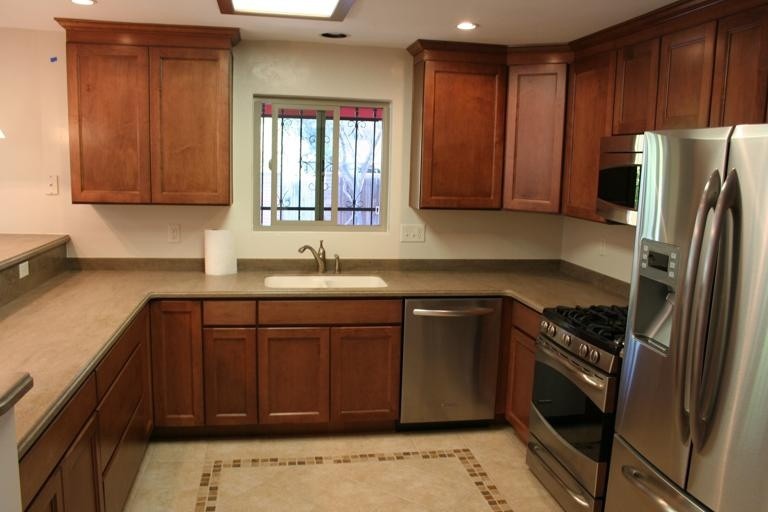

[537,301,627,376]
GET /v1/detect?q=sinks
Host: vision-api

[264,275,324,289]
[324,275,389,289]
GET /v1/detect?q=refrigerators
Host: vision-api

[597,121,765,512]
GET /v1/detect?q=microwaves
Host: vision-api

[596,131,646,228]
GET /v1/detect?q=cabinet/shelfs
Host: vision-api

[88,300,155,511]
[17,367,102,511]
[201,294,260,438]
[146,294,207,439]
[253,295,405,441]
[494,293,547,452]
[52,16,242,207]
[404,0,768,236]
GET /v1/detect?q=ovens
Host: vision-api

[519,341,619,511]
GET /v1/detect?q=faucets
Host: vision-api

[298,239,326,274]
